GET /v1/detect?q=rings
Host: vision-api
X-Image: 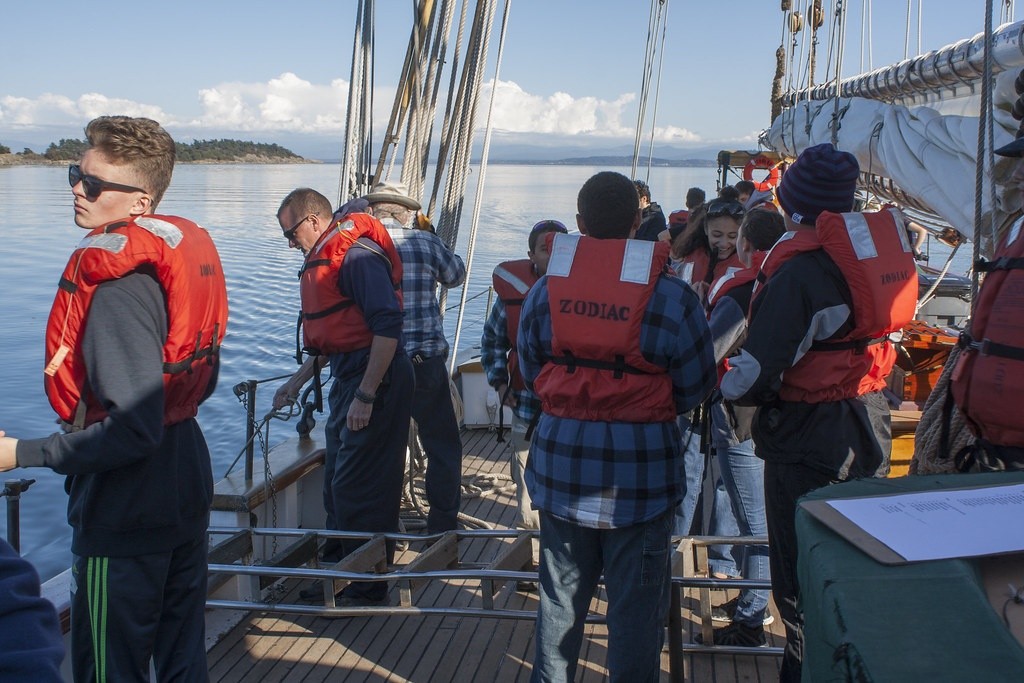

[359,425,363,426]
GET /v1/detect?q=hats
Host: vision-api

[993,132,1024,158]
[775,143,861,226]
[361,181,422,210]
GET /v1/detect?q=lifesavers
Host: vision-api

[743,155,779,192]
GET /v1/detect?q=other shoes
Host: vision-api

[427,535,466,547]
[318,588,392,619]
[712,572,728,580]
[516,563,542,591]
[299,577,324,602]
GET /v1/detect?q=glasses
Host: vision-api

[533,219,568,233]
[707,202,746,218]
[68,163,154,207]
[283,212,320,241]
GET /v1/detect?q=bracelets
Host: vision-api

[354,388,375,403]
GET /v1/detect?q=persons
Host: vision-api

[0,117,228,683]
[273,188,415,619]
[0,537,66,683]
[517,171,717,683]
[867,200,927,258]
[362,182,465,546]
[720,143,918,574]
[480,220,568,592]
[635,180,780,591]
[692,209,787,646]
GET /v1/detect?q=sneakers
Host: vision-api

[712,597,776,626]
[691,620,770,647]
